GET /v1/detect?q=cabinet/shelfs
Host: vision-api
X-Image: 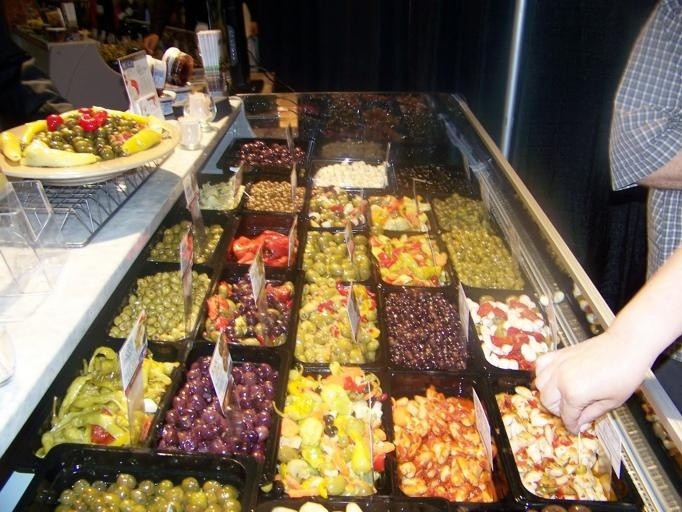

[13,25,157,114]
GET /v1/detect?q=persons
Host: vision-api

[534,244,682,436]
[608,0,682,415]
[144,0,206,56]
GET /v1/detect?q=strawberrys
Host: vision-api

[95,111,107,126]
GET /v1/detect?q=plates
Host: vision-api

[0,105,181,187]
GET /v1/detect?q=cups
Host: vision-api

[177,90,219,151]
[31,24,67,42]
